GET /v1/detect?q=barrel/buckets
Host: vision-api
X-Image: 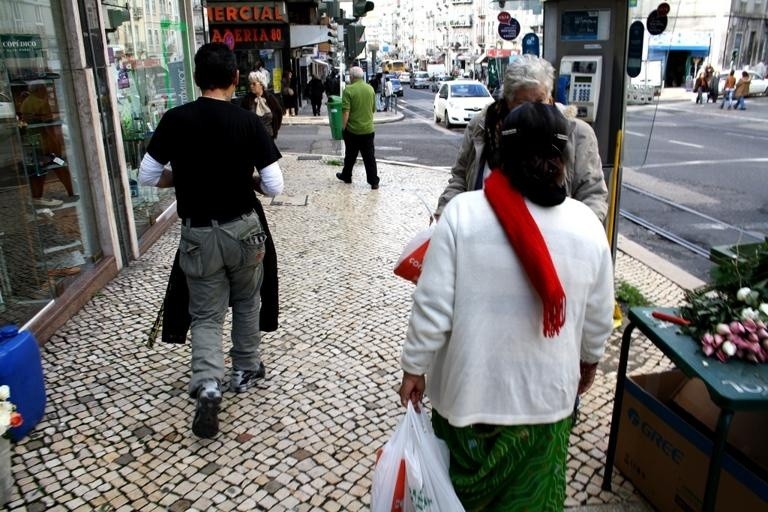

[0,325,46,442]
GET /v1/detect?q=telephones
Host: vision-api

[554,54,604,124]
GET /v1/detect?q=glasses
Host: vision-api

[250,82,261,87]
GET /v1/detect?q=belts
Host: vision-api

[181,210,254,229]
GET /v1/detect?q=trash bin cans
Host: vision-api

[324,95,343,142]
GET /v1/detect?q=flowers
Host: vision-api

[0,385,24,436]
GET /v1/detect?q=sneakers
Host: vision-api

[192,382,222,440]
[372,184,379,189]
[230,361,266,393]
[336,172,352,183]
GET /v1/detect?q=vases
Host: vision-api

[0,436,12,505]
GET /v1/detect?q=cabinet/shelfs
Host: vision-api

[10,78,84,287]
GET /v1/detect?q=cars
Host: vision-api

[384,71,430,97]
[432,75,496,128]
[718,70,768,97]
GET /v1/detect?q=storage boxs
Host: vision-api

[613,370,768,512]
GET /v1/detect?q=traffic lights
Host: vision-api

[353,1,374,17]
[347,24,366,59]
[318,0,340,17]
[327,23,338,66]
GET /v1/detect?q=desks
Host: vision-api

[600,307,768,512]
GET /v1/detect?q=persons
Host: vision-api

[282,62,393,116]
[434,54,609,223]
[398,101,615,512]
[693,64,751,110]
[137,40,285,438]
[20,80,80,202]
[239,70,284,142]
[336,66,380,189]
